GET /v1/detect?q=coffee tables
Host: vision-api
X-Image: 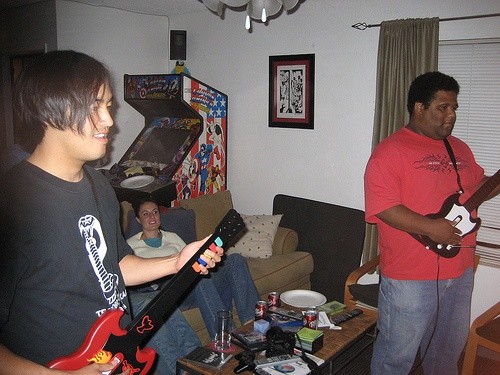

[176,302,378,375]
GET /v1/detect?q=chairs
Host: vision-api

[461,301,500,375]
[344,253,480,305]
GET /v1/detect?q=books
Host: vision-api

[182,345,233,370]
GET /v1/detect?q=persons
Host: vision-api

[1,48,224,375]
[121,198,264,375]
[364,71,500,375]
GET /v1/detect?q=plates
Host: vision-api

[120,175,154,189]
[280,289,327,309]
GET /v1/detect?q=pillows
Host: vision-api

[126,208,197,245]
[226,214,284,259]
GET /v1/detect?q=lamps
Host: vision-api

[201,0,299,30]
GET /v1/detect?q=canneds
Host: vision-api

[268,291,280,307]
[302,310,317,330]
[254,300,268,321]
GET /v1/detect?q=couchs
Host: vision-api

[121,190,314,345]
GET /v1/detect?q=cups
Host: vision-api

[214,310,231,351]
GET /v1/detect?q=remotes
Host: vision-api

[332,308,363,326]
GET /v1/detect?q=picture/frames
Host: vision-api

[267,53,316,130]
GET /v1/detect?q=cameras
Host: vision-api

[265,326,295,358]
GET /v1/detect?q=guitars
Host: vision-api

[415,169,499,258]
[48,208,248,375]
